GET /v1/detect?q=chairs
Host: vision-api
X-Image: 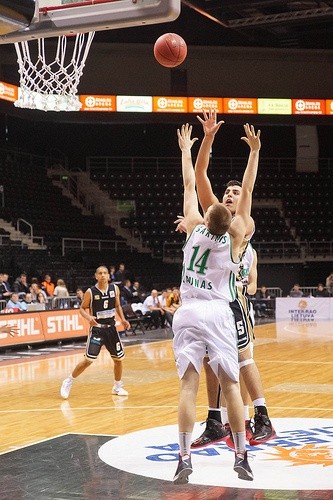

[0,164,333,336]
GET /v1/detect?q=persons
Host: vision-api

[0,324,20,337]
[0,272,69,311]
[74,288,83,308]
[290,283,304,297]
[257,286,275,317]
[171,108,278,484]
[313,274,333,297]
[110,264,180,327]
[60,267,130,400]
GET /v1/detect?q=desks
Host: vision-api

[0,308,126,353]
[275,297,333,321]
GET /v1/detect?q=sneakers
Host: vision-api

[173,451,193,485]
[190,418,230,449]
[249,414,276,446]
[233,450,254,481]
[60,378,73,400]
[112,386,128,396]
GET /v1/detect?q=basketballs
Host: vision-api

[154,33,187,67]
